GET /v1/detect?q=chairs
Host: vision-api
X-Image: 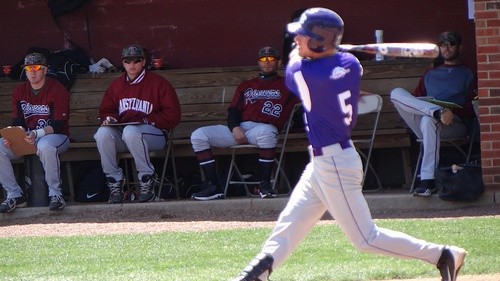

[410,100,481,194]
[352,90,384,194]
[115,124,182,201]
[223,103,303,197]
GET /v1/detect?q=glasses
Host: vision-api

[258,56,277,62]
[124,57,142,63]
[24,65,45,72]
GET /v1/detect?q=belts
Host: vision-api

[313,140,351,156]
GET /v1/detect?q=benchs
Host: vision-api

[0,58,429,207]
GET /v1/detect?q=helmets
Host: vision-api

[286,7,345,53]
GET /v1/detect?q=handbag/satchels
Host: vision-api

[45,57,75,92]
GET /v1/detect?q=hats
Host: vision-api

[24,52,48,67]
[434,31,461,46]
[259,47,280,59]
[121,44,144,59]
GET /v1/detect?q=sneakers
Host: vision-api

[257,180,276,198]
[190,185,224,200]
[0,196,29,213]
[435,244,468,281]
[138,172,157,202]
[105,174,126,204]
[48,194,66,210]
[414,179,438,196]
[439,108,455,127]
[232,254,274,281]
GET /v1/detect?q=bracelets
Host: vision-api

[33,129,46,138]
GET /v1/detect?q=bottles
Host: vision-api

[375,29,384,61]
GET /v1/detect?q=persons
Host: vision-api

[94,44,181,203]
[191,47,373,200]
[391,32,477,195]
[0,53,71,213]
[236,7,468,281]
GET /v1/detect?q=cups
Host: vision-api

[154,59,162,69]
[3,66,11,76]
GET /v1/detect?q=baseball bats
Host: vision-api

[292,42,439,58]
